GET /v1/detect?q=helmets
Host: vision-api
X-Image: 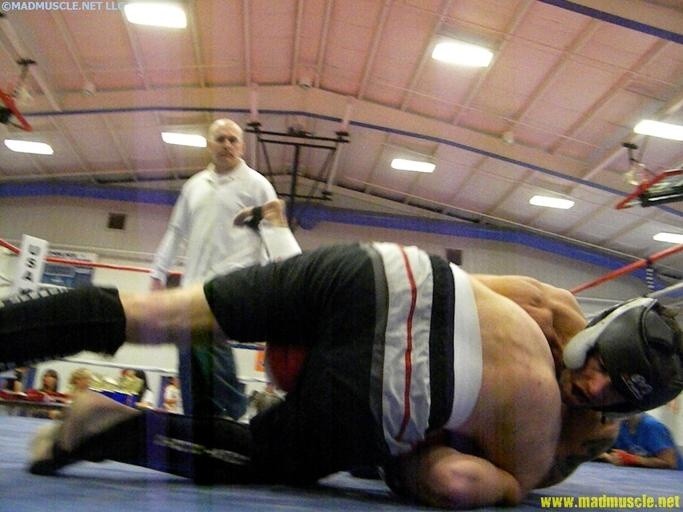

[563,296,683,414]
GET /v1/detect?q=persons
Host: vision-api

[119,367,147,402]
[247,382,284,415]
[57,198,683,509]
[0,240,563,501]
[148,118,278,422]
[590,411,683,471]
[158,376,185,416]
[6,366,28,393]
[31,369,63,419]
